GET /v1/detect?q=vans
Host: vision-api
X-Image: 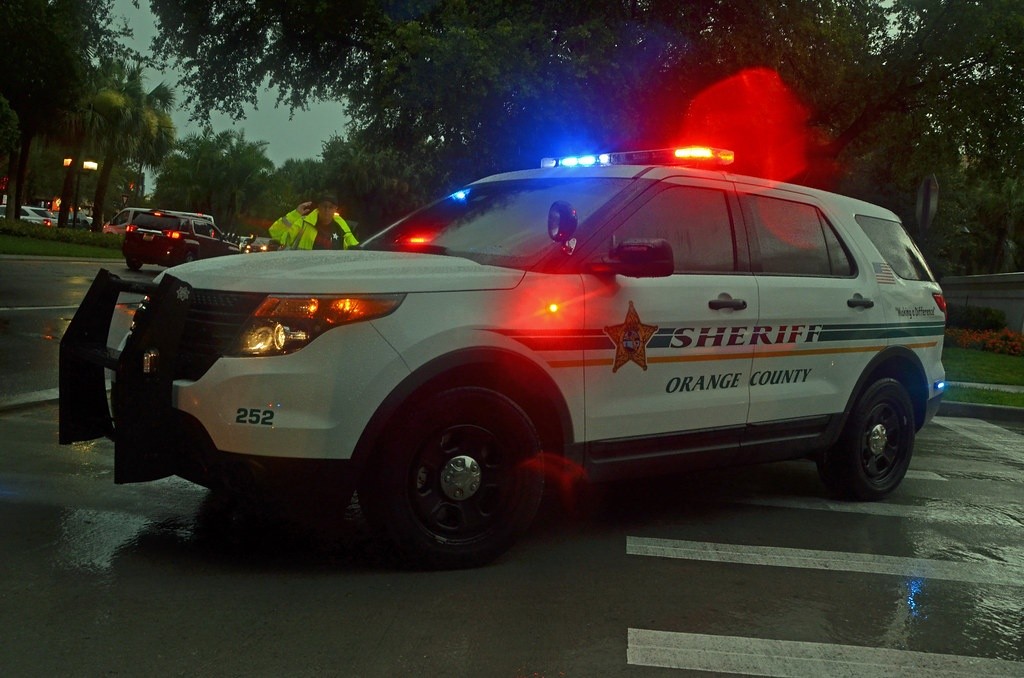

[102,208,214,236]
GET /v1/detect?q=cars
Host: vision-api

[0,204,58,227]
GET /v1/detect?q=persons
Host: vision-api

[268,193,360,251]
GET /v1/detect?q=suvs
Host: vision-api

[122,212,240,271]
[59,146,947,571]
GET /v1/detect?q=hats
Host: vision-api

[315,191,338,206]
